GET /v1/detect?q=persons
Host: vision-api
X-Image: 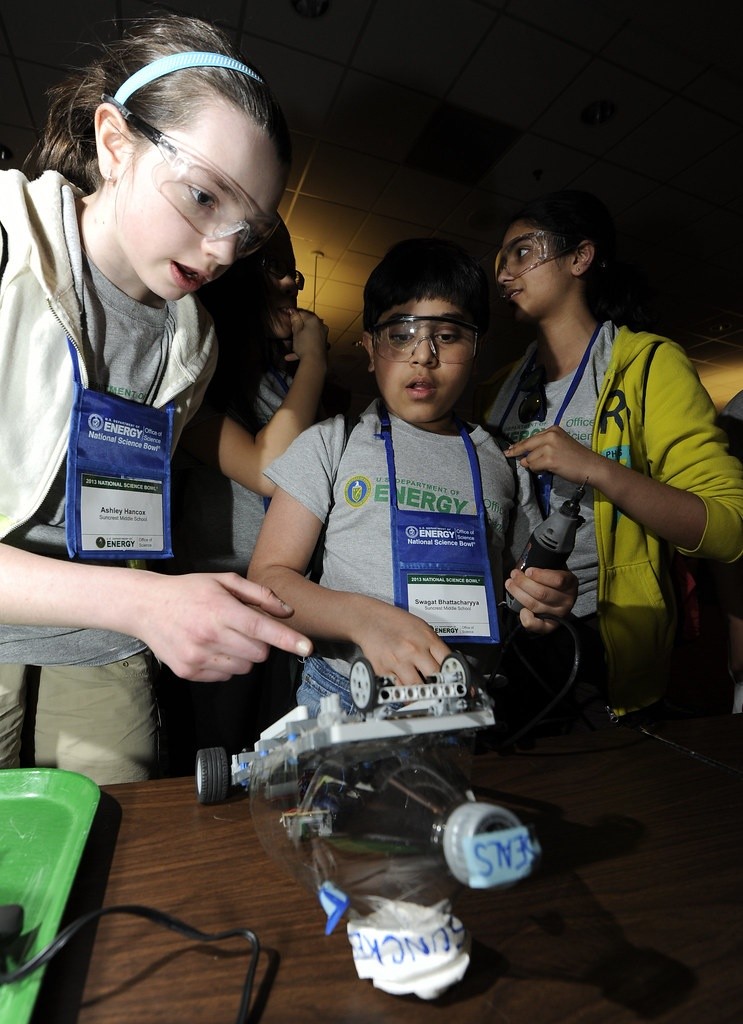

[0,9,314,686]
[162,199,743,694]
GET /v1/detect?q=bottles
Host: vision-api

[249,738,529,915]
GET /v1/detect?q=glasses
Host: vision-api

[250,255,305,292]
[517,364,547,424]
[494,230,578,279]
[350,315,482,364]
[100,92,286,259]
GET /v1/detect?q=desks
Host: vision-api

[0,714,743,1024]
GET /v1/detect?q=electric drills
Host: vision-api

[505,474,591,617]
